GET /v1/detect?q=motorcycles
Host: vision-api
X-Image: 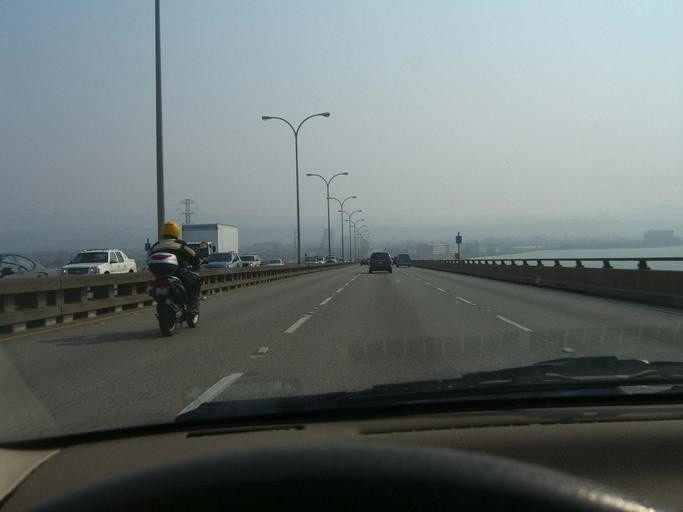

[351,224,373,261]
[144,241,210,336]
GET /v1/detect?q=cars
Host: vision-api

[0,252,57,281]
[265,258,284,266]
[358,256,398,266]
[302,254,343,264]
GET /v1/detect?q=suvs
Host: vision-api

[238,254,261,268]
[202,250,243,269]
[367,251,392,274]
[395,253,411,268]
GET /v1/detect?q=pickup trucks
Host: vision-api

[58,247,136,277]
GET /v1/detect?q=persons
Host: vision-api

[149,220,204,312]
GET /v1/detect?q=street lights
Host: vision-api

[324,194,357,261]
[344,218,364,262]
[260,111,332,263]
[304,171,349,262]
[455,236,462,244]
[336,208,361,261]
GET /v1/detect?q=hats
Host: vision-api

[163,221,180,237]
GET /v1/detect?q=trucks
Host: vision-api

[181,222,238,257]
